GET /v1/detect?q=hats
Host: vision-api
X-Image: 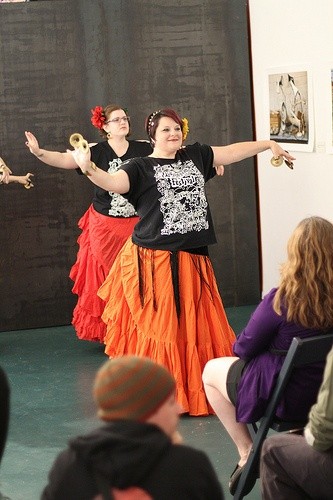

[92,355,177,423]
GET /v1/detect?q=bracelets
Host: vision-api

[83,162,97,177]
[36,149,46,158]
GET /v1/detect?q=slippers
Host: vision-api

[228,463,261,499]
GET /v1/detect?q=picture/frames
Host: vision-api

[268,69,315,153]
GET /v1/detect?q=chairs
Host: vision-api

[232,334,333,500]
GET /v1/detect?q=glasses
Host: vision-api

[104,116,130,125]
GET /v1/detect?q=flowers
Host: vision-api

[180,118,190,141]
[89,105,105,129]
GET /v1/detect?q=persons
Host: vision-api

[39,355,225,500]
[260,347,333,500]
[0,157,35,187]
[202,216,333,496]
[65,107,297,416]
[25,105,224,343]
